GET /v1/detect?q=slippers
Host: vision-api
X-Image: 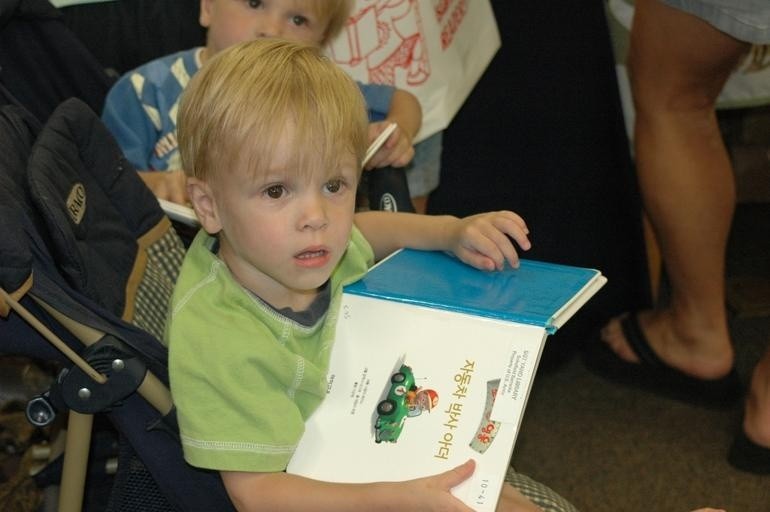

[728,419,769,474]
[602,310,738,404]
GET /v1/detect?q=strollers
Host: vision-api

[1,69,584,512]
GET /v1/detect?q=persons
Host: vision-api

[97,0,422,248]
[162,39,580,512]
[583,0,769,476]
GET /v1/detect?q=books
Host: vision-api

[155,118,399,229]
[283,245,612,512]
